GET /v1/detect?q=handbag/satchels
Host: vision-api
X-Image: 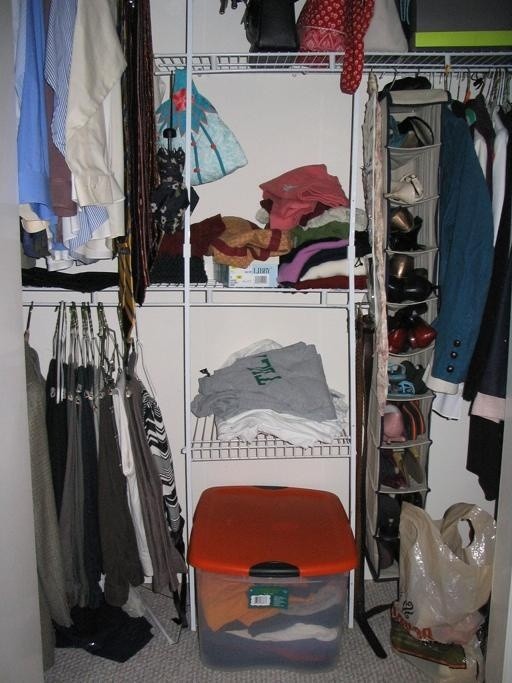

[390,500,497,683]
[297,1,374,67]
[155,69,248,185]
[241,0,298,68]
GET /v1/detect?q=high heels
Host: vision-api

[384,173,424,206]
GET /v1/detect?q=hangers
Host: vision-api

[365,63,511,132]
[22,298,161,410]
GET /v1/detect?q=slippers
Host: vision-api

[388,360,429,395]
[381,447,423,490]
[399,400,425,440]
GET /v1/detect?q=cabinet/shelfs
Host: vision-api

[17,50,510,632]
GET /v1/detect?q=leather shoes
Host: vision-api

[388,208,424,252]
[388,311,437,354]
[387,254,432,302]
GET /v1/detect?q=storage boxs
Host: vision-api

[184,484,362,674]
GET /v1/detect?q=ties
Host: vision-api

[112,1,160,342]
